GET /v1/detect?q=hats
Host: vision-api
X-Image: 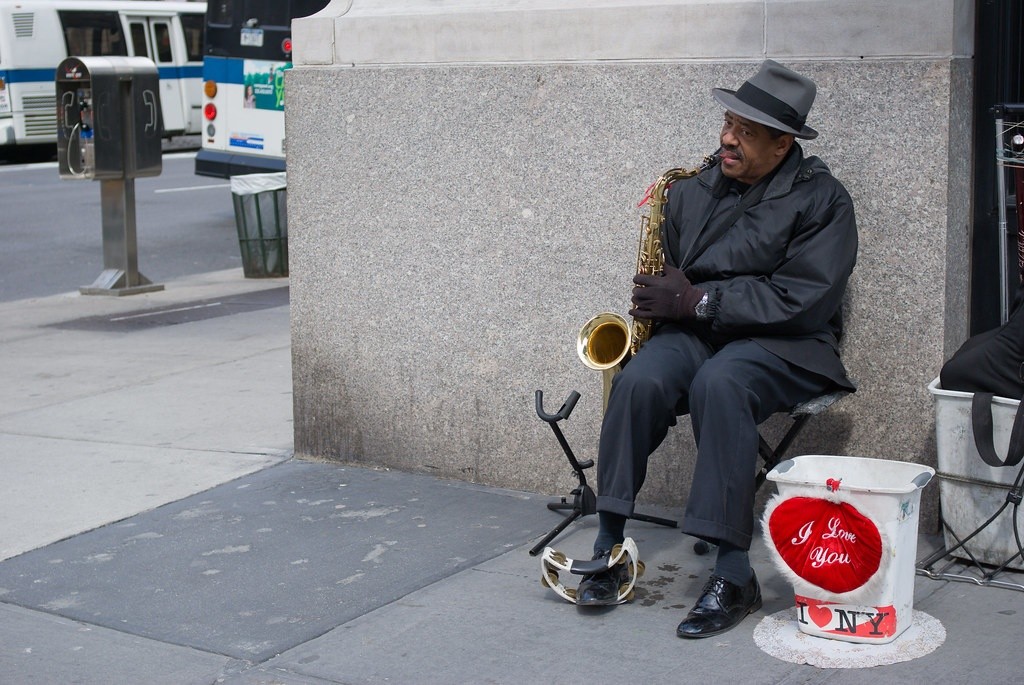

[712,59,819,140]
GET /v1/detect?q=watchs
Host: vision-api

[694,293,708,320]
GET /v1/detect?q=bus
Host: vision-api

[0,0,210,161]
[193,0,332,183]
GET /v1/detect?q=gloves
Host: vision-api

[628,262,707,322]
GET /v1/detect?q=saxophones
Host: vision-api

[575,153,725,418]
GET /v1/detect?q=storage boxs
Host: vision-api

[928,374,1024,571]
[766,455,936,645]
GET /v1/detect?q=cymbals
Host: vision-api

[539,537,646,606]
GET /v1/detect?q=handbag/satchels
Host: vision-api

[941,308,1024,467]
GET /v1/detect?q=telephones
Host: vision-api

[78,97,94,141]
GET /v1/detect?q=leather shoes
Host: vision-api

[675,566,763,638]
[574,547,630,605]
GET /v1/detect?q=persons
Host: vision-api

[576,57,858,638]
[244,86,257,109]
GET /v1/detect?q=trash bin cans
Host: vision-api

[232,172,289,279]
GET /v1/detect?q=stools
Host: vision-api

[694,390,850,556]
